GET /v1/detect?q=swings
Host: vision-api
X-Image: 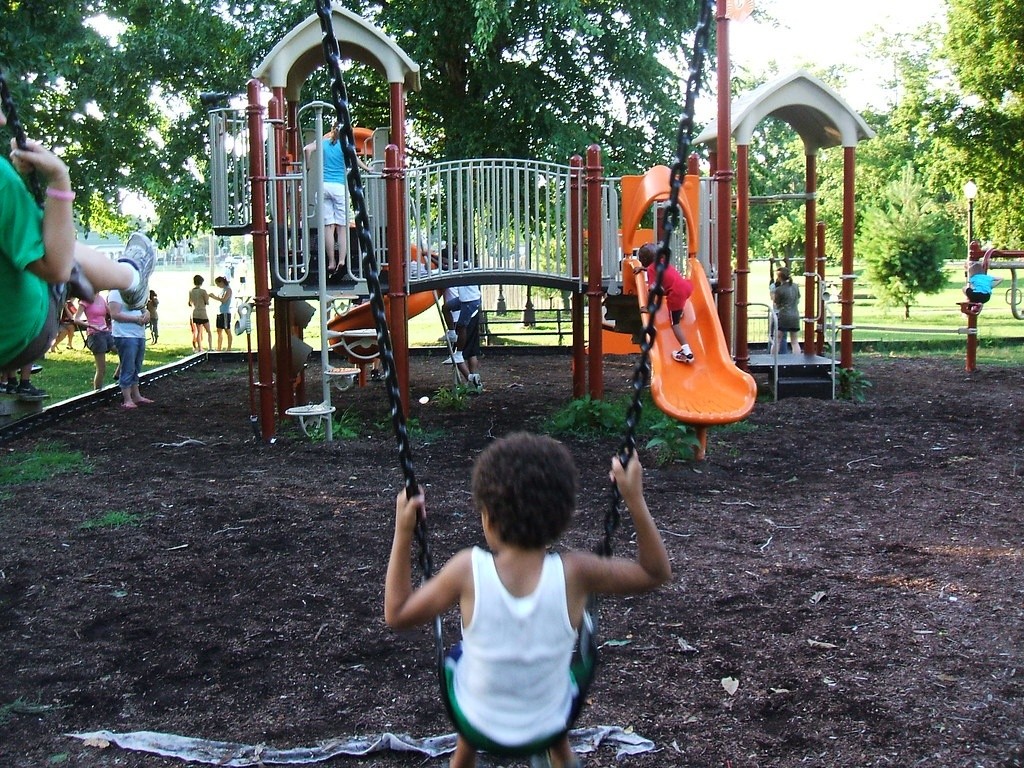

[315,0,714,759]
[0,68,95,313]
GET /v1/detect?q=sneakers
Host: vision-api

[117,231,155,309]
[437,334,457,342]
[6,382,19,393]
[470,373,484,395]
[17,383,47,396]
[671,349,694,363]
[441,352,464,365]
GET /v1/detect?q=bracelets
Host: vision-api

[46,188,76,201]
[136,315,141,325]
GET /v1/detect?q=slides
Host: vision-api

[326,241,444,365]
[624,257,758,426]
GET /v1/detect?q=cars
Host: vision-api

[158,253,243,264]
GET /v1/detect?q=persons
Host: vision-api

[422,242,483,389]
[0,363,49,398]
[302,122,373,272]
[962,264,1003,312]
[347,295,383,383]
[383,432,671,768]
[188,257,248,352]
[51,285,160,409]
[769,267,800,354]
[0,107,157,373]
[632,243,694,363]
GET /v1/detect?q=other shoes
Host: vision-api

[17,364,43,374]
[134,398,154,404]
[120,402,137,410]
[327,265,335,271]
[973,302,981,312]
[0,381,8,392]
[337,263,346,276]
[371,368,382,382]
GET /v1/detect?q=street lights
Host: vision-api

[963,180,976,246]
